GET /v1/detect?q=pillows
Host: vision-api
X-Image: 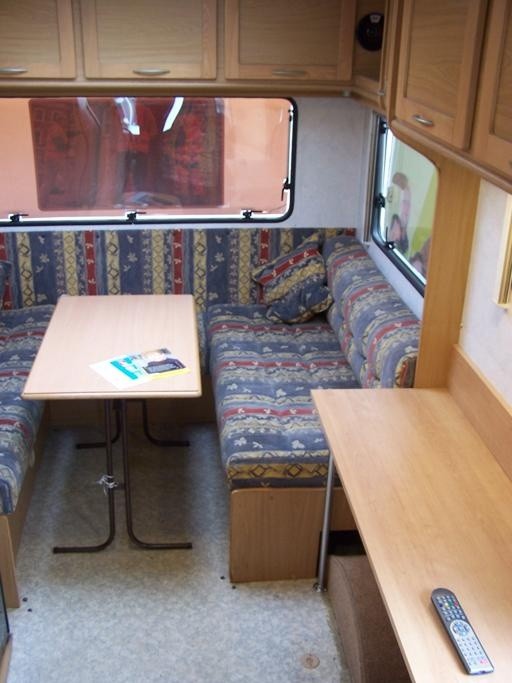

[0,258,15,314]
[250,240,336,325]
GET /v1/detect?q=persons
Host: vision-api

[141,348,180,374]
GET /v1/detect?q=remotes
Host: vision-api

[432,587,494,675]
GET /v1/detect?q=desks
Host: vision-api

[21,295,203,553]
[310,385,512,683]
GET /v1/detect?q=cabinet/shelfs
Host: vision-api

[393,0,512,186]
[224,0,357,80]
[0,0,76,79]
[80,0,218,80]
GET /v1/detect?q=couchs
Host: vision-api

[0,228,422,609]
